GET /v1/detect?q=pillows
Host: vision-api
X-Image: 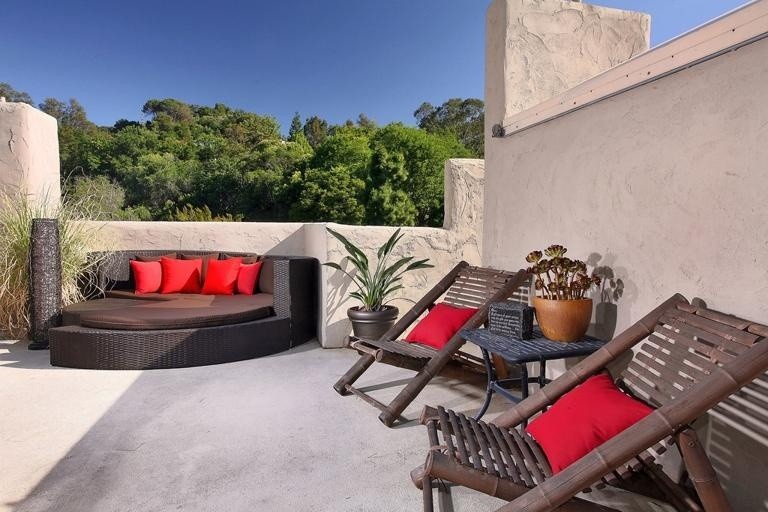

[128,252,273,297]
[526,372,655,475]
[402,299,478,351]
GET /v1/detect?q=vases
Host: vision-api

[533,294,595,344]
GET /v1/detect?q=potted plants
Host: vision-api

[319,224,436,356]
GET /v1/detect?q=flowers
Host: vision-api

[525,244,601,302]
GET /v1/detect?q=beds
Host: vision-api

[48,249,319,369]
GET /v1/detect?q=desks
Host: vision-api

[462,324,607,438]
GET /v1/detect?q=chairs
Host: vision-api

[408,293,767,511]
[331,260,531,427]
